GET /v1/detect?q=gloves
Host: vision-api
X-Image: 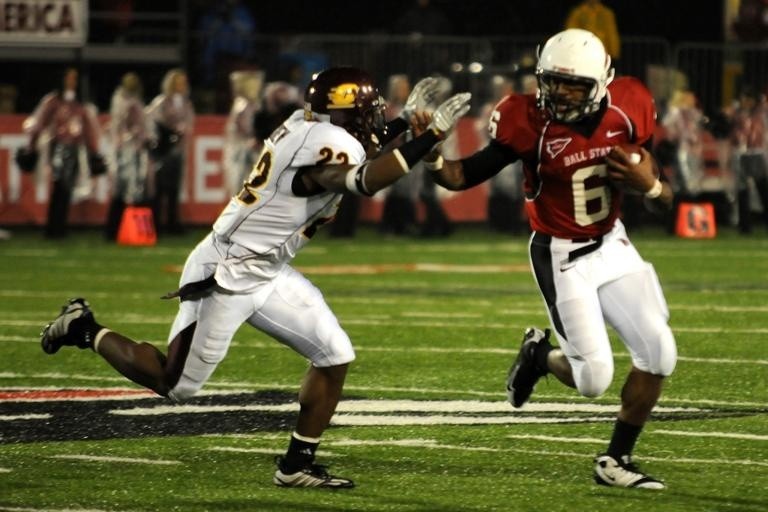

[399,77,443,124]
[427,93,471,139]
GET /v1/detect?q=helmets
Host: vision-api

[537,28,614,123]
[306,66,389,154]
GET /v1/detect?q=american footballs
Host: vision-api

[606,143,661,198]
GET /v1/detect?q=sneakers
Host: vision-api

[272,455,352,488]
[506,327,551,407]
[41,298,92,354]
[592,456,664,491]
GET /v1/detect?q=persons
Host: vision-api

[40,64,472,489]
[403,28,677,492]
